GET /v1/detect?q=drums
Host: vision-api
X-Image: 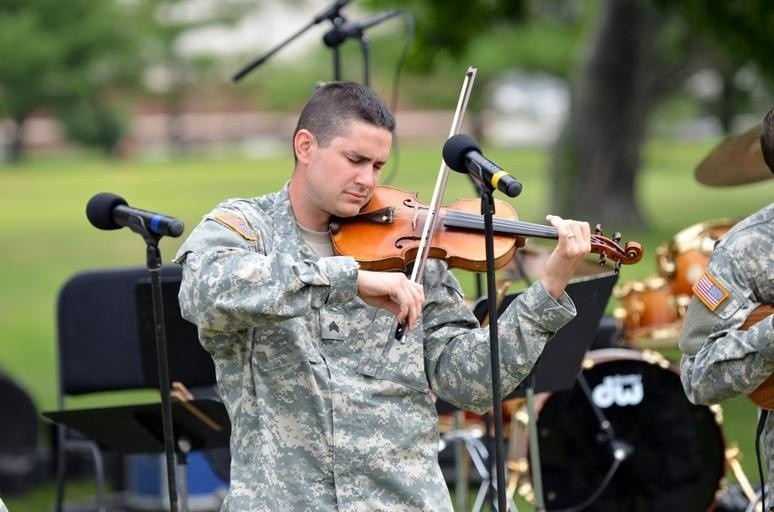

[436,399,524,438]
[517,348,731,512]
[615,279,682,341]
[651,219,737,315]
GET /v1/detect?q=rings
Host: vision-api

[568,232,575,240]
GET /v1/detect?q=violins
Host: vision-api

[329,186,642,276]
[735,305,774,408]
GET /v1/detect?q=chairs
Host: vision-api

[44,266,236,511]
[2,371,46,457]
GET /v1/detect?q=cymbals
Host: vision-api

[695,124,771,188]
[504,240,616,281]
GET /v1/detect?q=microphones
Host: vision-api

[322,12,391,46]
[85,192,182,240]
[442,132,525,199]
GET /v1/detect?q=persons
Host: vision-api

[174,78,593,512]
[679,104,774,512]
